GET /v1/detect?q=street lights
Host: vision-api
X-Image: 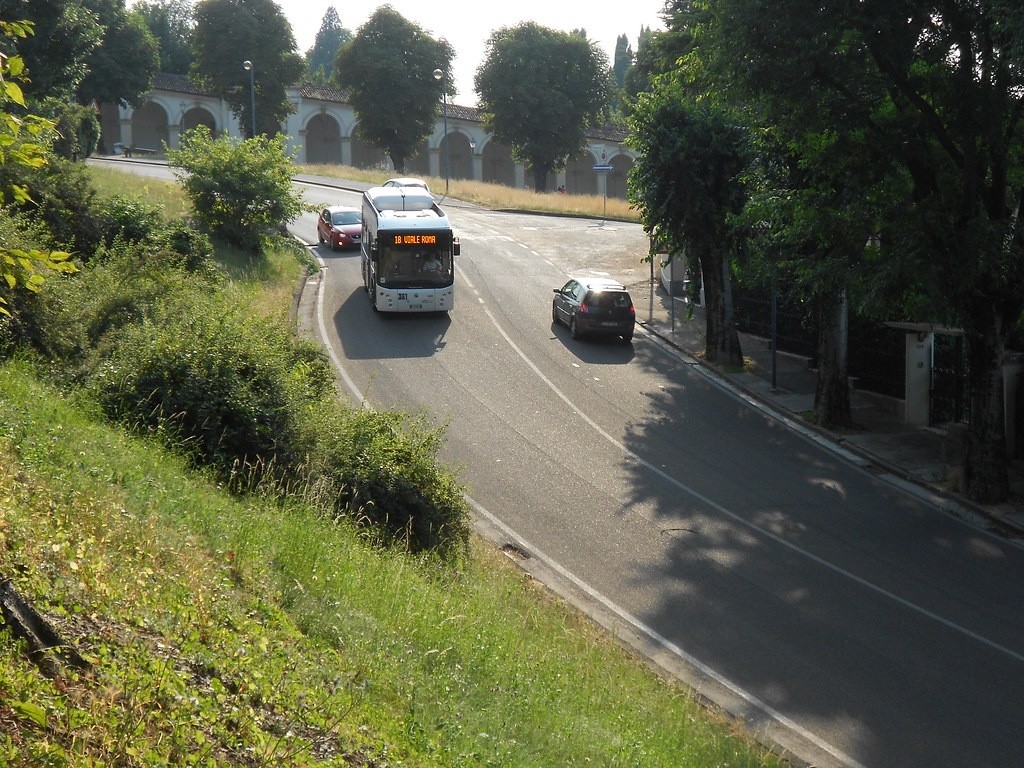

[243,60,255,139]
[433,69,449,194]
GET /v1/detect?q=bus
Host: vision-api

[361,187,460,312]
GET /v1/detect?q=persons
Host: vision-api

[557,186,566,193]
[424,255,442,273]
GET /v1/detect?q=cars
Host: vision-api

[552,278,636,342]
[317,207,362,250]
[381,178,431,195]
